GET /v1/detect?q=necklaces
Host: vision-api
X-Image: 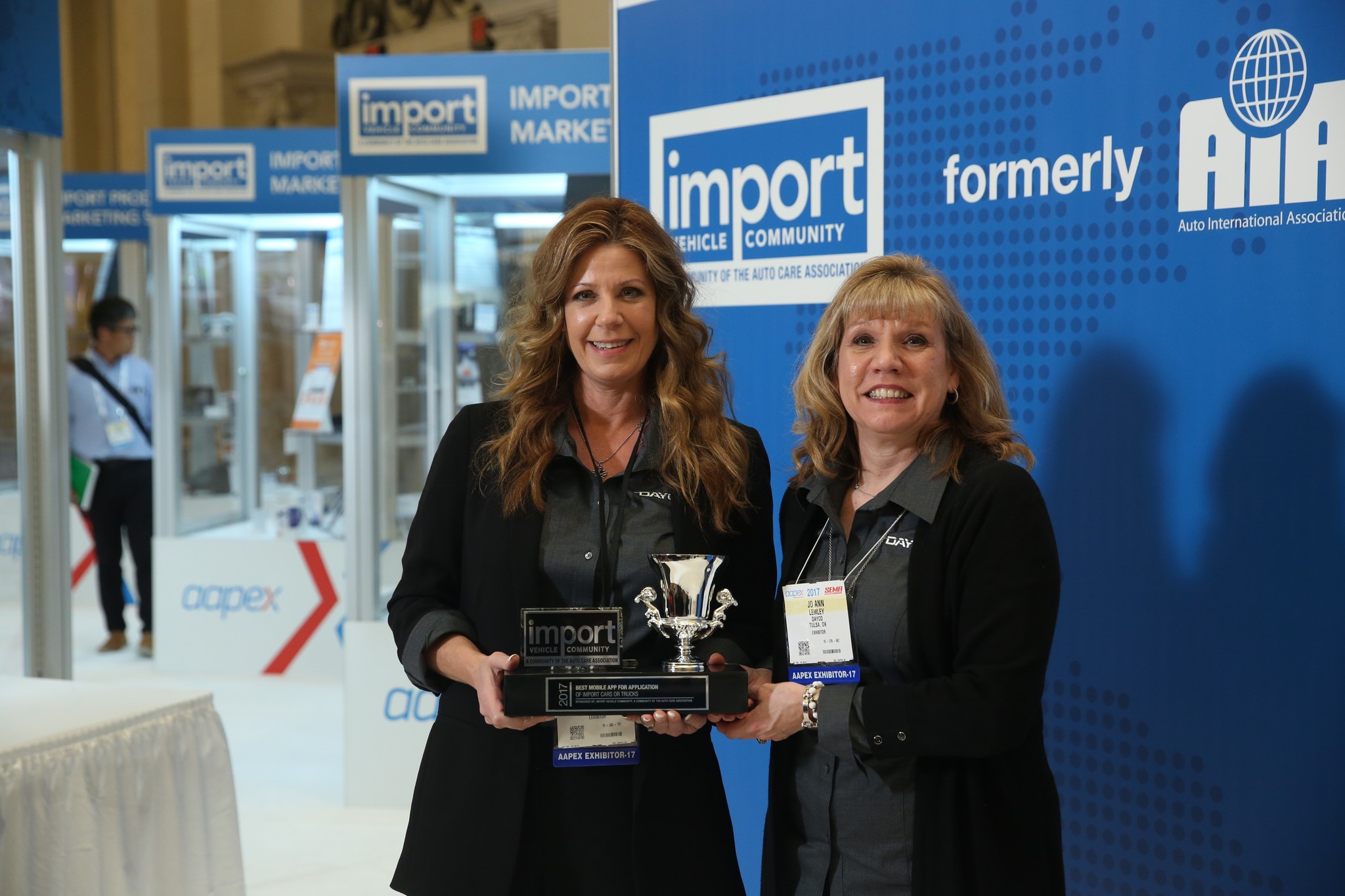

[853,472,880,498]
[568,401,648,479]
[828,510,903,600]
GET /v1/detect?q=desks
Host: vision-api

[0,669,251,896]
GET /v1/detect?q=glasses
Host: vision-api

[106,325,140,336]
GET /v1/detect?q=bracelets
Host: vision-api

[808,680,825,723]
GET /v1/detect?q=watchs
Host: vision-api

[802,681,818,731]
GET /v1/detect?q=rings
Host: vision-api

[756,738,768,745]
[642,723,654,732]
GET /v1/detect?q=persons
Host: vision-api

[387,195,777,896]
[66,297,154,655]
[709,253,1068,896]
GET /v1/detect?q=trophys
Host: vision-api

[634,553,739,672]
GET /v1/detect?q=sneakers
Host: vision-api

[100,630,127,652]
[139,629,153,658]
